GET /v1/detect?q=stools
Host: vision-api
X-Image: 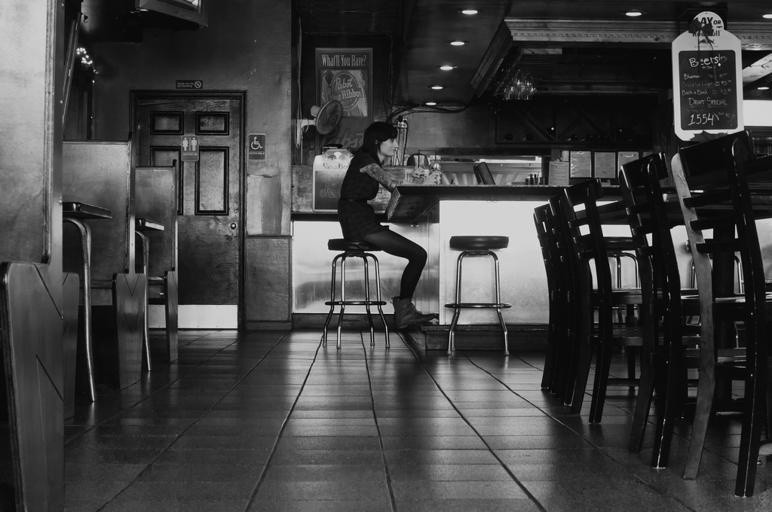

[322,236,390,352]
[441,235,512,360]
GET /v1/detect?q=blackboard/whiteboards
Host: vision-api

[672,11,744,142]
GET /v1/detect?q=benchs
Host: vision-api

[0,0,184,511]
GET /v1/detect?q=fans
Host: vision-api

[294,97,342,149]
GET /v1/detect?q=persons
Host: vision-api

[338,122,440,333]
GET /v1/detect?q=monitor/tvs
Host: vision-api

[113,0,208,27]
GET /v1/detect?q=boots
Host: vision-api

[391,295,438,333]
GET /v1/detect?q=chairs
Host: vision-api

[527,123,771,501]
